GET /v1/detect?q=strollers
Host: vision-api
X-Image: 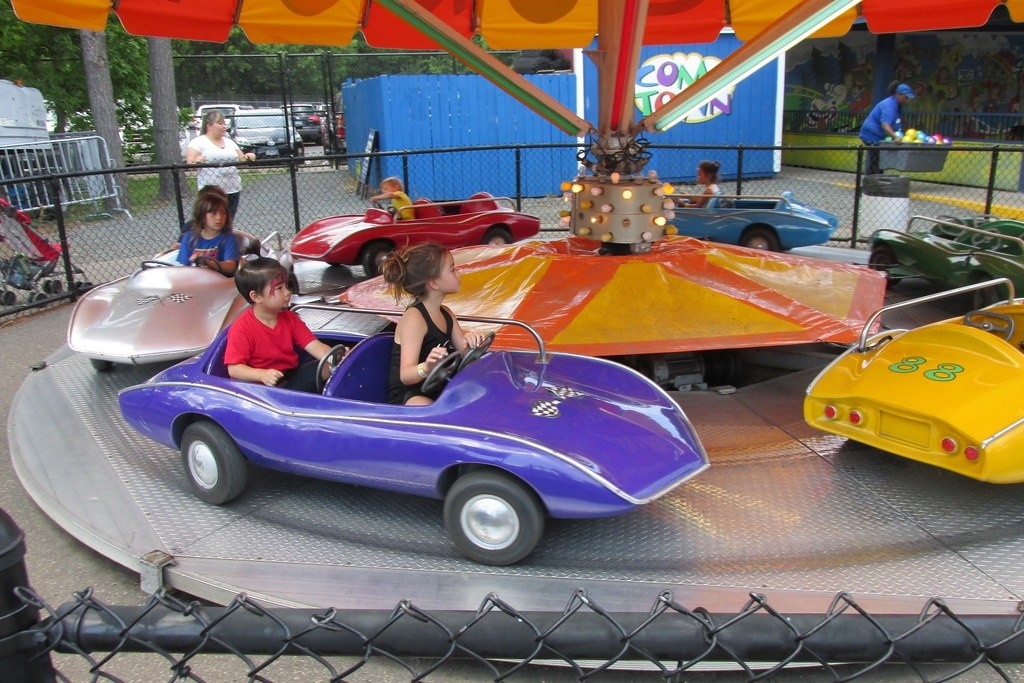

[0,196,94,309]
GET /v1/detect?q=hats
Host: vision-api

[896,84,914,99]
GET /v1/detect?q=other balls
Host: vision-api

[884,129,951,145]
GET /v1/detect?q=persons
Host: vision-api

[186,110,256,228]
[223,257,350,394]
[381,244,487,409]
[172,185,228,249]
[858,84,915,176]
[369,176,415,220]
[176,193,243,275]
[678,160,721,208]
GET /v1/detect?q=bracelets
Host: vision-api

[417,363,428,379]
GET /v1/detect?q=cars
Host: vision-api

[799,275,1024,487]
[289,192,542,281]
[65,228,294,373]
[664,191,840,253]
[188,100,347,167]
[868,214,1024,309]
[118,301,711,567]
[116,107,190,164]
